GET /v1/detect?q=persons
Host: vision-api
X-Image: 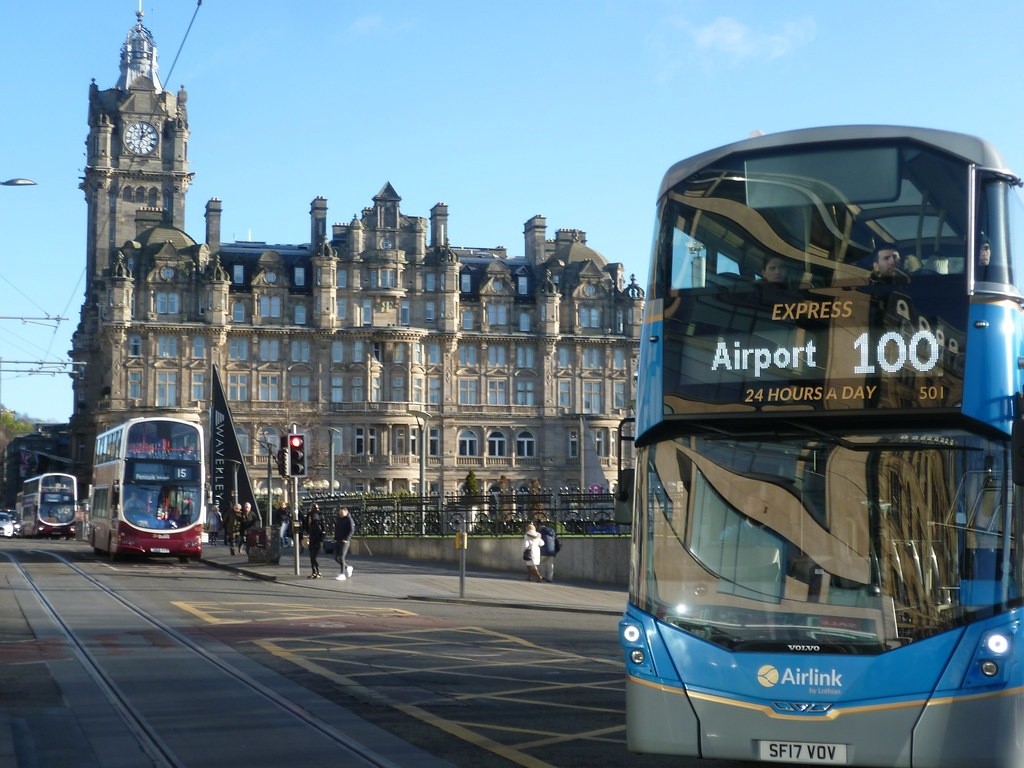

[206,505,223,546]
[924,255,948,275]
[753,254,789,290]
[976,228,992,268]
[521,522,545,582]
[872,244,902,278]
[332,507,355,580]
[276,501,291,544]
[306,503,326,534]
[536,520,560,582]
[223,501,259,556]
[298,508,305,555]
[124,491,146,510]
[306,518,324,580]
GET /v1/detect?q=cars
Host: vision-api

[0,512,13,539]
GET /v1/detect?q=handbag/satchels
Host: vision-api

[555,538,563,554]
[522,548,532,561]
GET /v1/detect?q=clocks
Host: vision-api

[123,120,160,157]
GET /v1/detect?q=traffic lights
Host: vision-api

[288,433,308,477]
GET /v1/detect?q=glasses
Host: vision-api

[976,245,990,253]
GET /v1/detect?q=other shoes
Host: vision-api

[526,576,532,581]
[230,549,236,556]
[238,545,242,553]
[333,574,347,581]
[536,576,543,583]
[542,576,553,583]
[346,566,353,577]
[306,573,322,579]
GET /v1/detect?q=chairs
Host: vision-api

[706,272,742,289]
[126,451,199,461]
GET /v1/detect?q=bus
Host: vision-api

[20,473,78,540]
[88,417,212,562]
[619,125,1024,768]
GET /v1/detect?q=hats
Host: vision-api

[525,524,536,531]
[964,229,991,247]
[923,254,949,274]
[903,254,923,273]
